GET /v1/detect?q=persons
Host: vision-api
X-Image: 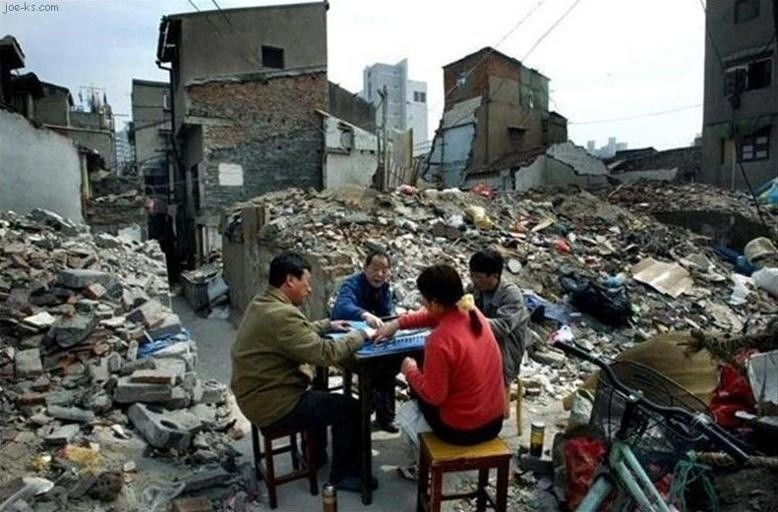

[330,248,399,433]
[461,247,528,387]
[231,250,378,493]
[369,263,505,484]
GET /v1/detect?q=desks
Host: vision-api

[317,315,424,506]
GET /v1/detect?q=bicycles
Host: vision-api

[547,339,765,512]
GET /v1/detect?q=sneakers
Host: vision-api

[396,462,432,487]
[376,418,399,431]
[330,475,378,491]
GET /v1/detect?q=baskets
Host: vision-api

[588,357,717,484]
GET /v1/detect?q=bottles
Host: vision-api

[321,482,337,512]
[530,420,546,457]
